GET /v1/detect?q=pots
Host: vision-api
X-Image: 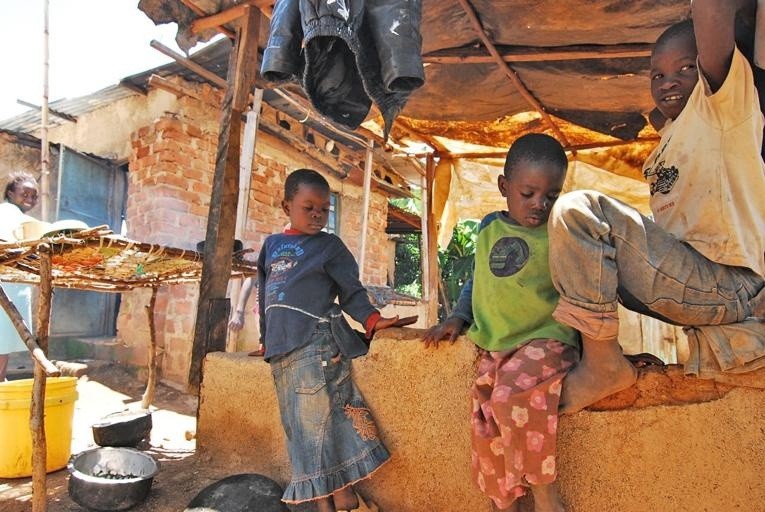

[197,238,254,260]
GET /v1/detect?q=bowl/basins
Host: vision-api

[43,219,90,237]
[67,447,161,511]
[91,409,153,447]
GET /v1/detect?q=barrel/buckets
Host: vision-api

[0,376,79,479]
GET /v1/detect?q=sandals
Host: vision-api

[337,491,379,512]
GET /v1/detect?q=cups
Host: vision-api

[13,220,41,239]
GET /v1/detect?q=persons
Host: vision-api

[546,0,765,417]
[421,133,582,512]
[227,264,258,334]
[0,172,54,382]
[249,168,419,512]
